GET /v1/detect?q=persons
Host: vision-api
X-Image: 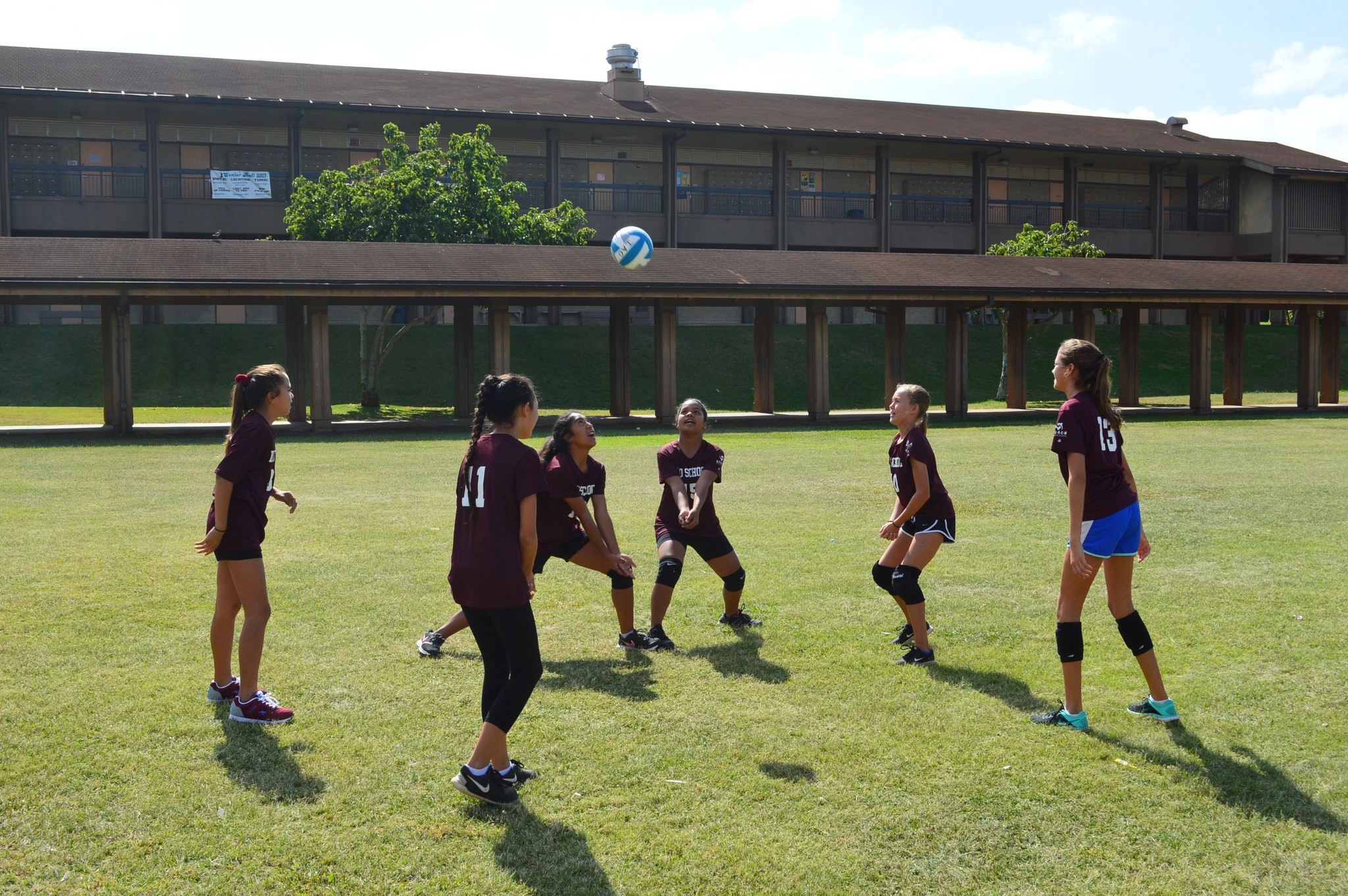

[871,386,956,666]
[194,367,298,725]
[1028,338,1180,729]
[646,399,763,649]
[416,407,660,656]
[447,372,542,805]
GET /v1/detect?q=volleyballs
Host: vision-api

[610,226,654,270]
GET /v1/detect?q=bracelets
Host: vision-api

[888,521,893,522]
[214,527,226,532]
[893,522,901,528]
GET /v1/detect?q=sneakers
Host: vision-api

[451,764,519,805]
[647,625,675,647]
[207,675,268,704]
[417,630,445,659]
[1127,694,1179,722]
[502,759,537,788]
[229,688,294,724]
[1028,706,1088,731]
[717,609,763,628]
[890,621,933,646]
[893,643,936,664]
[616,629,661,650]
